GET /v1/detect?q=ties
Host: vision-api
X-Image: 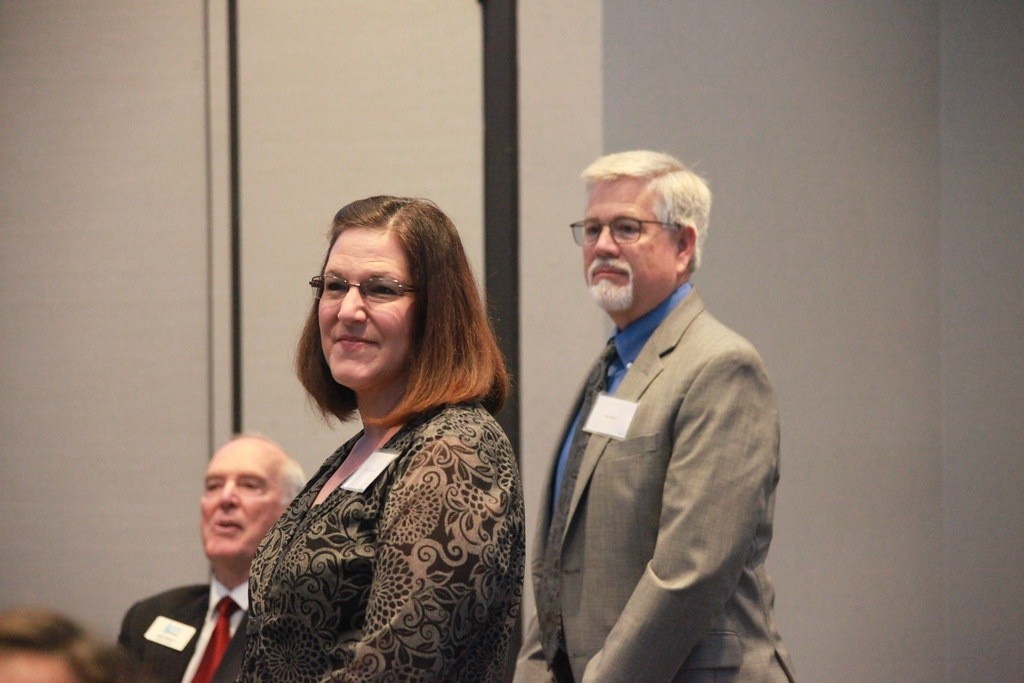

[189,595,240,683]
[538,337,619,672]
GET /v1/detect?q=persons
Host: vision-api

[118,430,312,683]
[236,191,525,683]
[532,147,799,682]
[0,608,160,683]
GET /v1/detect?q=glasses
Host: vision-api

[569,217,683,247]
[309,274,422,301]
